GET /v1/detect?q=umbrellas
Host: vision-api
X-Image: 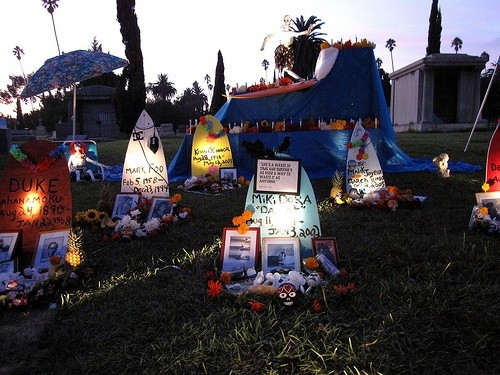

[20,50,129,141]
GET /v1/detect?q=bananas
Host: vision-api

[336,198,345,204]
[101,214,118,228]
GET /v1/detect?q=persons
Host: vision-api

[67,141,108,182]
[120,197,130,215]
[0,264,10,274]
[317,242,334,263]
[40,242,60,263]
[226,174,231,179]
[279,248,287,264]
[152,201,170,220]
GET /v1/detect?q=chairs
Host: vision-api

[34,125,51,141]
[157,123,174,138]
[64,140,106,181]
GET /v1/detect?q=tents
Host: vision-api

[165,41,414,173]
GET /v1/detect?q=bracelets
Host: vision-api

[96,161,98,166]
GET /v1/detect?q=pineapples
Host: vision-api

[97,184,113,213]
[330,170,345,198]
[65,228,85,267]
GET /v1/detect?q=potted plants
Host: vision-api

[321,116,324,122]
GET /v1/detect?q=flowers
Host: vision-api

[231,77,293,95]
[232,209,253,234]
[320,38,377,49]
[184,115,381,134]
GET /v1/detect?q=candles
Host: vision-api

[330,118,332,122]
[334,118,335,121]
[290,117,292,124]
[374,117,378,128]
[300,117,303,128]
[233,122,237,127]
[340,38,343,47]
[229,122,231,130]
[283,118,286,131]
[256,122,259,132]
[356,37,357,44]
[331,40,333,46]
[194,118,198,126]
[241,118,244,128]
[317,115,321,127]
[272,121,275,130]
[189,119,192,134]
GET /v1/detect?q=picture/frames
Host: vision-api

[112,192,142,221]
[0,228,23,263]
[31,227,72,270]
[219,226,260,274]
[263,236,301,277]
[218,166,238,183]
[0,257,19,282]
[147,195,173,222]
[475,191,500,221]
[311,235,341,263]
